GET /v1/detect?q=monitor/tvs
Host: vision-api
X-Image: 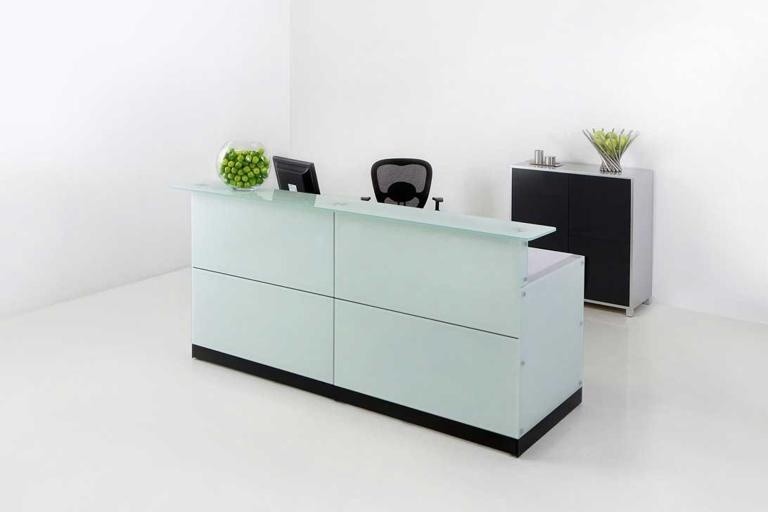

[273,156,320,194]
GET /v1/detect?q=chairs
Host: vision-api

[360,156,444,215]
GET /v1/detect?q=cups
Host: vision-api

[534,150,543,165]
[544,155,552,166]
[552,156,560,166]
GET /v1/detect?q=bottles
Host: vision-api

[214,135,275,192]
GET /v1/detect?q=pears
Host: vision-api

[592,128,628,159]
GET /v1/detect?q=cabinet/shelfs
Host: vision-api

[509,160,654,318]
